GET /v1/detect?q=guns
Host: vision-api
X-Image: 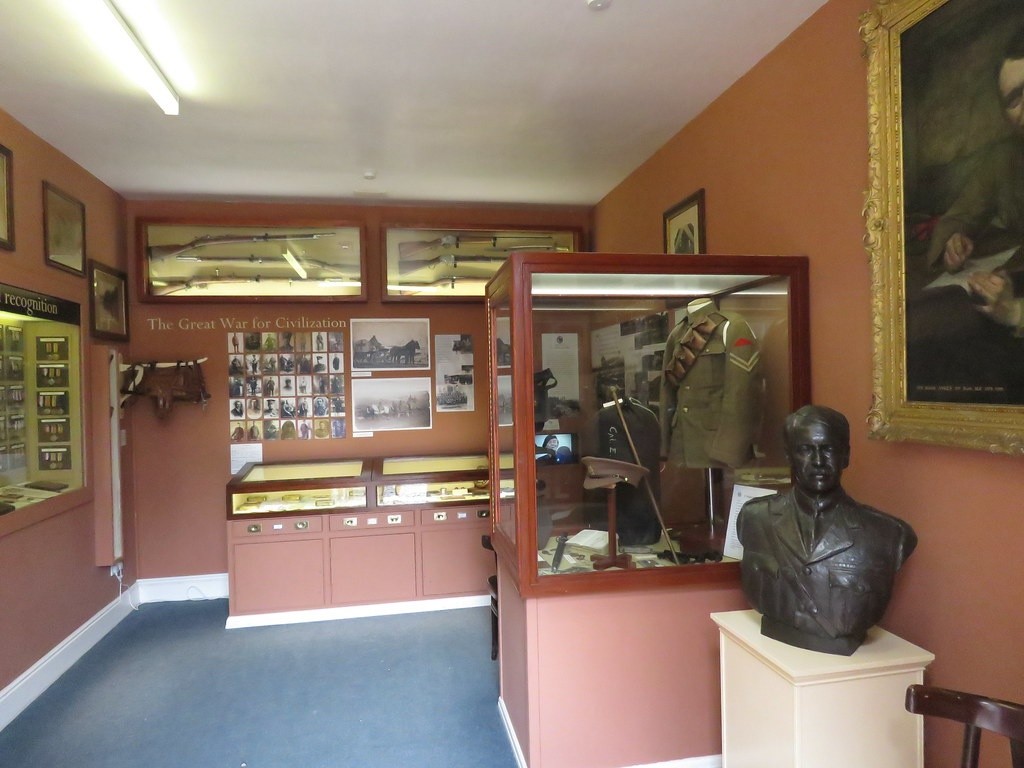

[147,231,360,293]
[396,234,557,296]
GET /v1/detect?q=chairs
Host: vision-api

[906,682,1024,768]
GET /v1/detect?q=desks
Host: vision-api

[710,608,936,768]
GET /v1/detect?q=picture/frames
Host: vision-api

[0,144,16,252]
[662,187,707,254]
[86,258,131,343]
[350,318,432,372]
[857,0,1024,458]
[351,377,433,433]
[40,179,88,278]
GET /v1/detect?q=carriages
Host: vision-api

[353,336,420,367]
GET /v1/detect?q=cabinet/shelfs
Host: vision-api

[223,450,547,631]
[484,253,812,768]
[378,221,585,303]
[136,214,372,306]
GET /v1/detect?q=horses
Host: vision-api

[390,340,420,365]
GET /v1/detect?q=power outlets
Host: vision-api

[110,561,123,576]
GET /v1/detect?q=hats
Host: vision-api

[582,456,650,491]
[317,356,323,360]
[282,332,292,338]
[267,400,275,404]
[542,434,557,448]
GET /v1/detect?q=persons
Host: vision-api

[657,296,763,470]
[229,332,346,442]
[542,434,568,465]
[737,405,918,657]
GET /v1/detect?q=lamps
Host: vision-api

[66,0,181,116]
